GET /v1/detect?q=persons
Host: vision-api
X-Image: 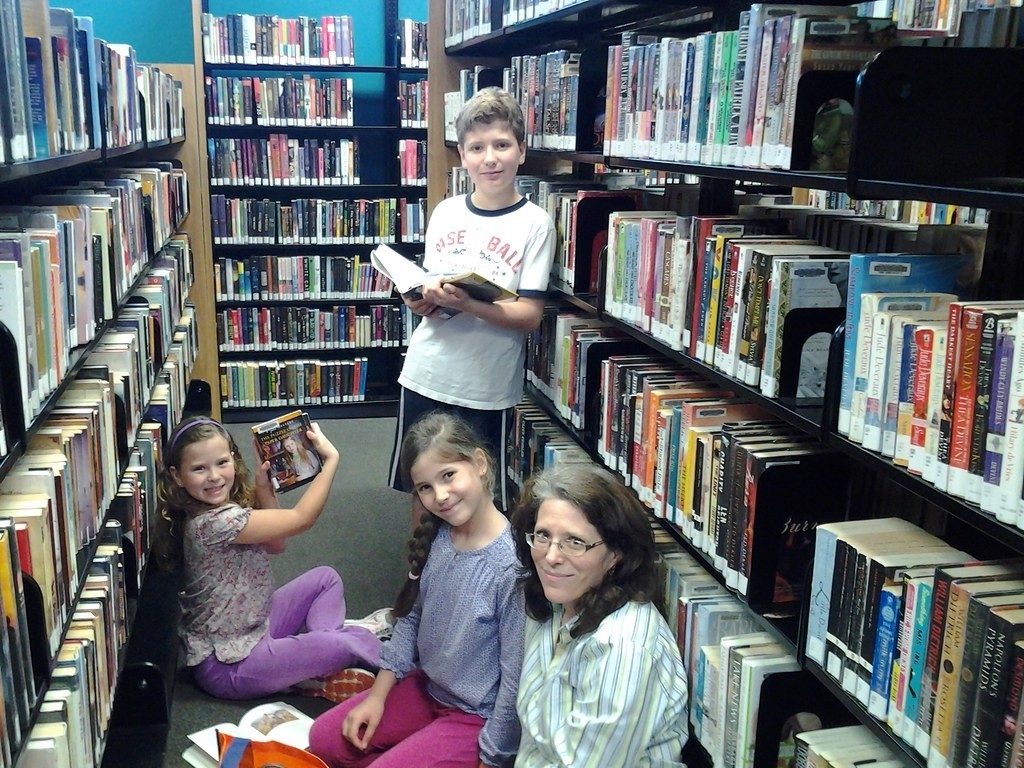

[282,436,320,481]
[157,416,397,702]
[389,87,557,526]
[824,262,850,307]
[308,413,526,768]
[515,463,688,768]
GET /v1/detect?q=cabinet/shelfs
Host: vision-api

[0,0,1024,768]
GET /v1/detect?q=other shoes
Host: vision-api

[291,667,376,706]
[343,607,397,640]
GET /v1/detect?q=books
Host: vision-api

[0,0,1024,768]
[252,410,322,494]
[182,701,314,768]
[370,243,517,320]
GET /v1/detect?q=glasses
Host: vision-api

[525,531,609,557]
